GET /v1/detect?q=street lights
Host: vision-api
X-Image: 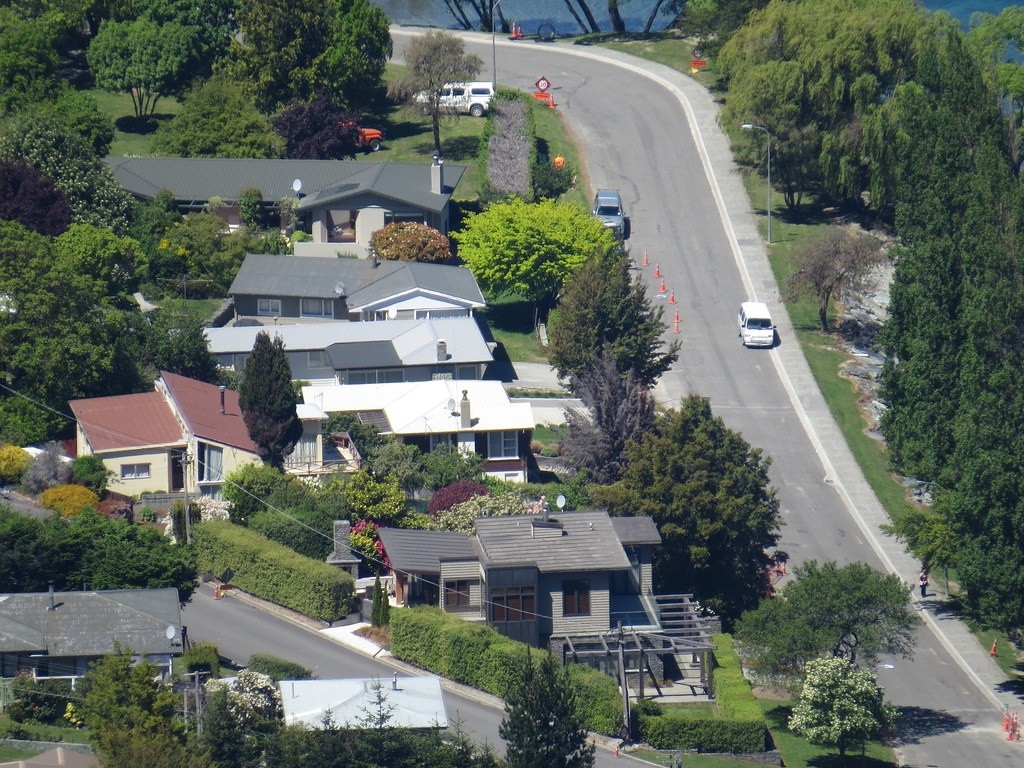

[742,124,771,242]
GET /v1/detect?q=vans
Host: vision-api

[738,302,777,346]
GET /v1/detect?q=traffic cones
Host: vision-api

[778,564,788,577]
[511,22,523,38]
[668,290,676,304]
[613,746,620,758]
[212,584,222,600]
[642,250,649,266]
[672,306,681,334]
[655,263,661,278]
[658,279,667,293]
[1003,707,1021,742]
[548,91,556,109]
[990,638,998,657]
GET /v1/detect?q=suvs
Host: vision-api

[338,121,382,152]
[413,81,496,117]
[591,191,625,235]
